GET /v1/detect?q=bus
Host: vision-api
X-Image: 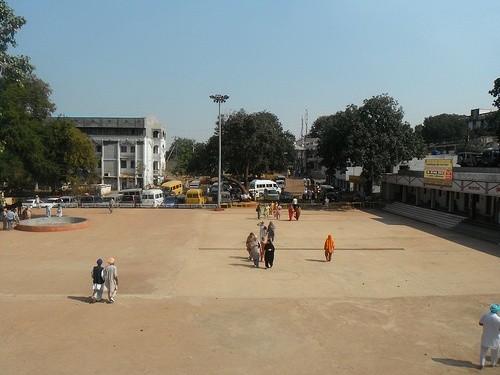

[118,188,142,203]
[79,194,118,209]
[160,179,183,198]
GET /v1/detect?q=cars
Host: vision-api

[58,195,75,208]
[456,140,500,169]
[21,199,44,208]
[40,197,60,208]
[189,177,242,203]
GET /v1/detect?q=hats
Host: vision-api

[108,258,115,264]
[490,304,499,313]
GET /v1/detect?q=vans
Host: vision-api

[275,175,285,190]
[140,188,164,208]
[185,189,209,208]
[320,184,338,202]
[248,179,279,201]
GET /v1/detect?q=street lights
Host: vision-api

[209,94,230,211]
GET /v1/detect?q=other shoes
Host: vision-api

[91,298,95,303]
[110,298,115,303]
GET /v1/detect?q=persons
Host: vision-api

[23,208,28,219]
[91,259,104,302]
[288,168,301,177]
[288,205,300,221]
[45,205,50,217]
[256,222,275,243]
[324,235,334,261]
[57,204,63,217]
[263,201,281,219]
[303,177,310,187]
[246,233,275,268]
[479,304,500,368]
[1,208,18,230]
[325,197,329,210]
[257,203,261,219]
[292,197,298,211]
[35,195,40,208]
[104,258,118,302]
[302,186,318,204]
[108,198,114,213]
[17,199,22,215]
